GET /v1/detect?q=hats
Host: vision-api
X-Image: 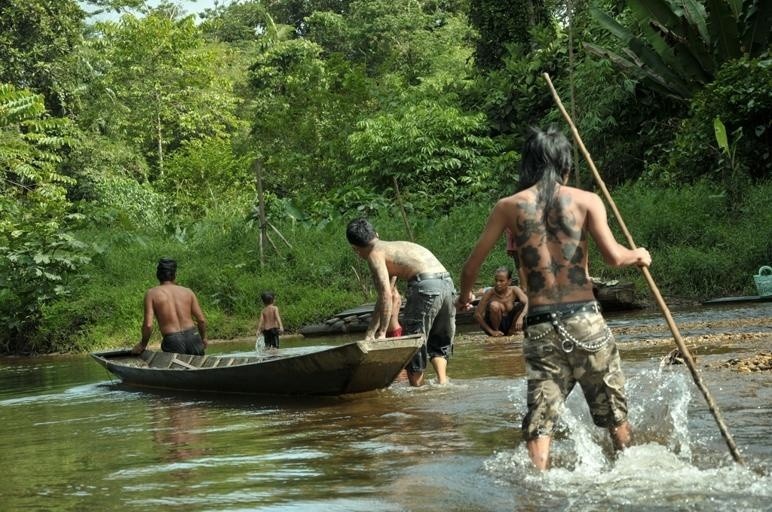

[157,258,176,272]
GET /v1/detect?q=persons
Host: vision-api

[345,217,458,392]
[386,274,403,337]
[131,258,208,357]
[453,128,654,476]
[254,291,286,352]
[504,222,530,297]
[473,266,529,338]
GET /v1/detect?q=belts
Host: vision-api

[522,302,602,329]
[408,271,451,283]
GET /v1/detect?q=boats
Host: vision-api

[90,332,424,392]
[296,279,638,338]
